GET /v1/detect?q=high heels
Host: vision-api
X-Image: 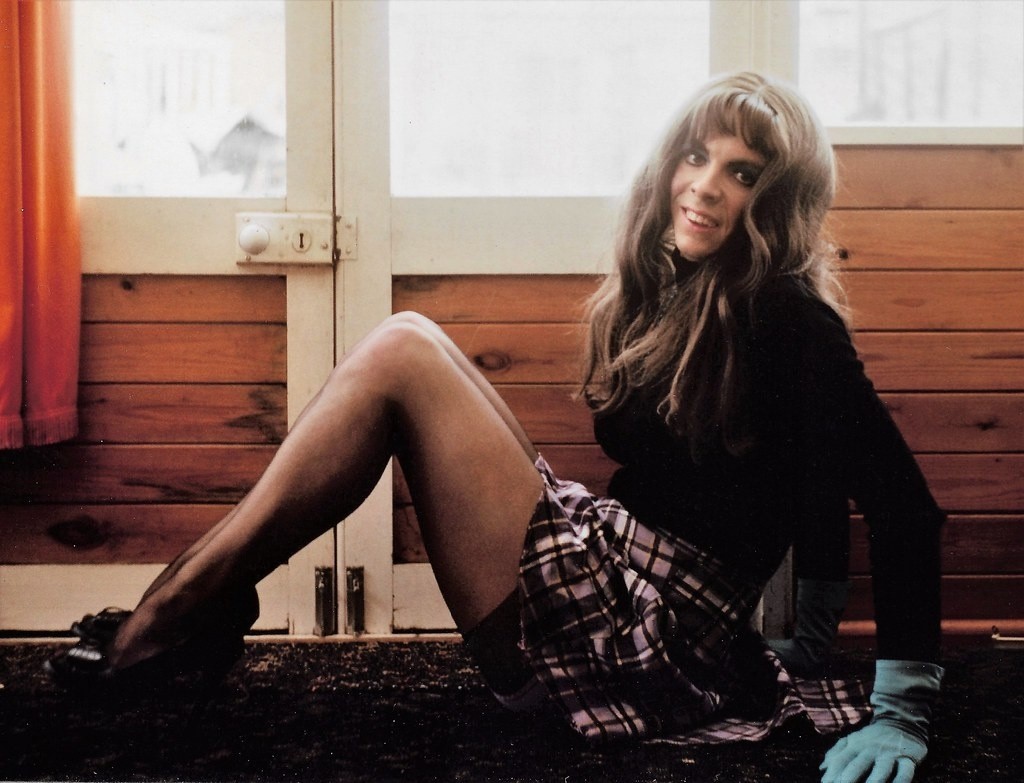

[0,584,260,723]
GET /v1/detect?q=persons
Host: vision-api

[45,73,946,783]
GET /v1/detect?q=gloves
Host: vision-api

[819,659,946,783]
